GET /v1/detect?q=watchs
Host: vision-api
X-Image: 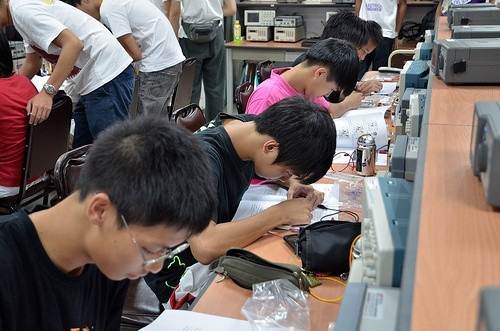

[43,83,58,95]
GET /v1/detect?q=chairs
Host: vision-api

[235,81,254,115]
[169,56,196,120]
[0,95,72,215]
[168,103,206,134]
[256,59,274,85]
[54,143,92,201]
[388,50,414,69]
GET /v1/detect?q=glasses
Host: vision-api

[93,192,190,266]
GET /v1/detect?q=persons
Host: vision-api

[435,0,496,28]
[0,29,40,199]
[0,0,136,149]
[62,0,187,123]
[0,116,218,331]
[290,10,383,104]
[355,0,406,82]
[143,97,336,310]
[150,0,237,122]
[244,39,359,186]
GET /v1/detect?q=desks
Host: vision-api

[223,37,417,117]
[187,71,401,331]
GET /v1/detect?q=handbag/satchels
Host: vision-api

[284,219,361,276]
[222,246,309,294]
[181,18,220,42]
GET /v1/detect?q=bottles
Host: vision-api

[234,20,241,44]
